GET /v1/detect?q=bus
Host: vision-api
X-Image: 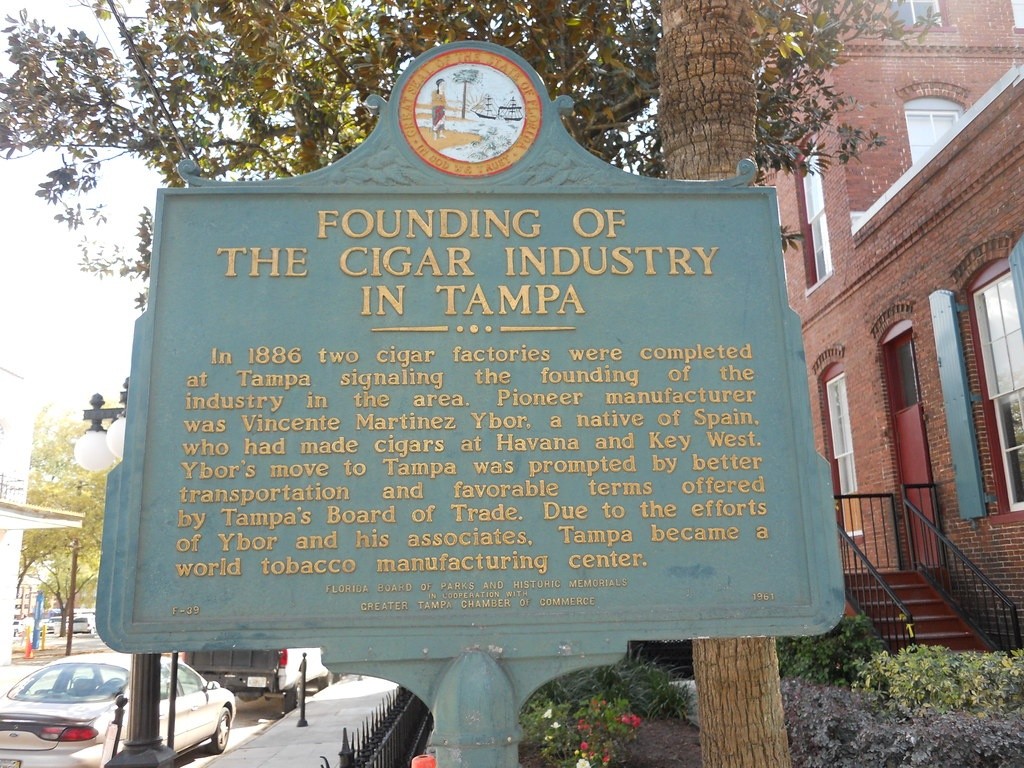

[45,607,96,616]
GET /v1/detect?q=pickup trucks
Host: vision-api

[182,646,342,717]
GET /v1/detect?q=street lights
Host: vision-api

[73,376,177,768]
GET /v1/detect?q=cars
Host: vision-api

[46,614,69,634]
[38,618,49,628]
[70,615,96,634]
[0,653,238,768]
[13,619,28,635]
